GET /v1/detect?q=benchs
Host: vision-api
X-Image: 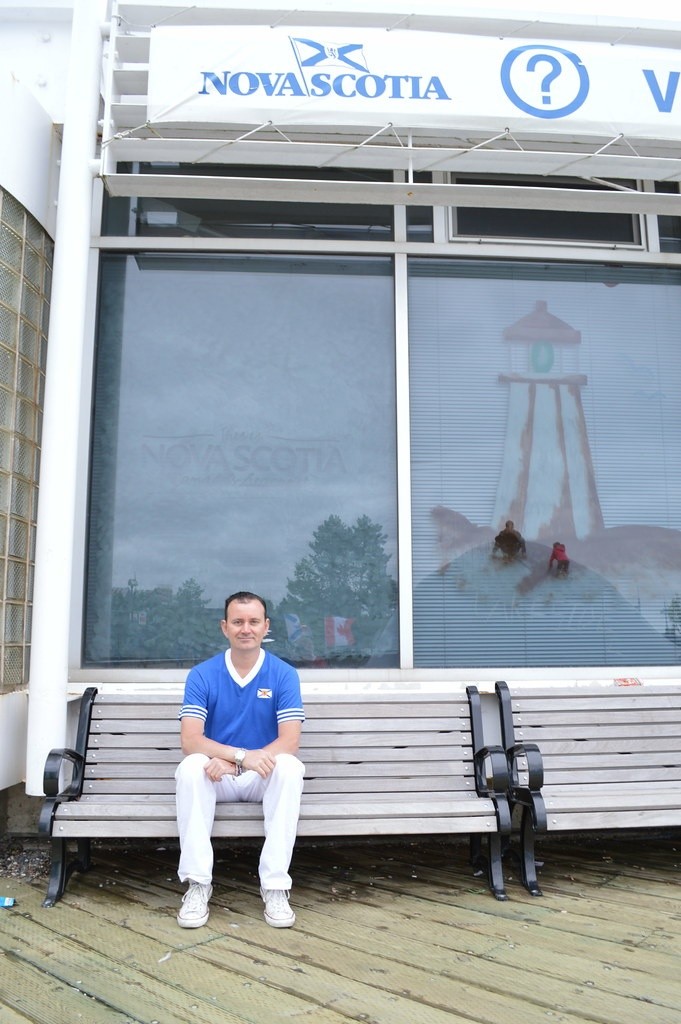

[495,680,681,896]
[38,685,512,908]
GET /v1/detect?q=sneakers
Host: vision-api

[260,886,296,928]
[177,878,213,930]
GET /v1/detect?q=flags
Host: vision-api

[324,615,356,647]
[284,612,304,644]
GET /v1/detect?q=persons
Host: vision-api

[174,592,305,927]
[491,521,526,559]
[548,541,572,577]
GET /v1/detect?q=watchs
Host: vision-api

[235,748,246,766]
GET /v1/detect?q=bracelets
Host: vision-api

[234,763,243,776]
